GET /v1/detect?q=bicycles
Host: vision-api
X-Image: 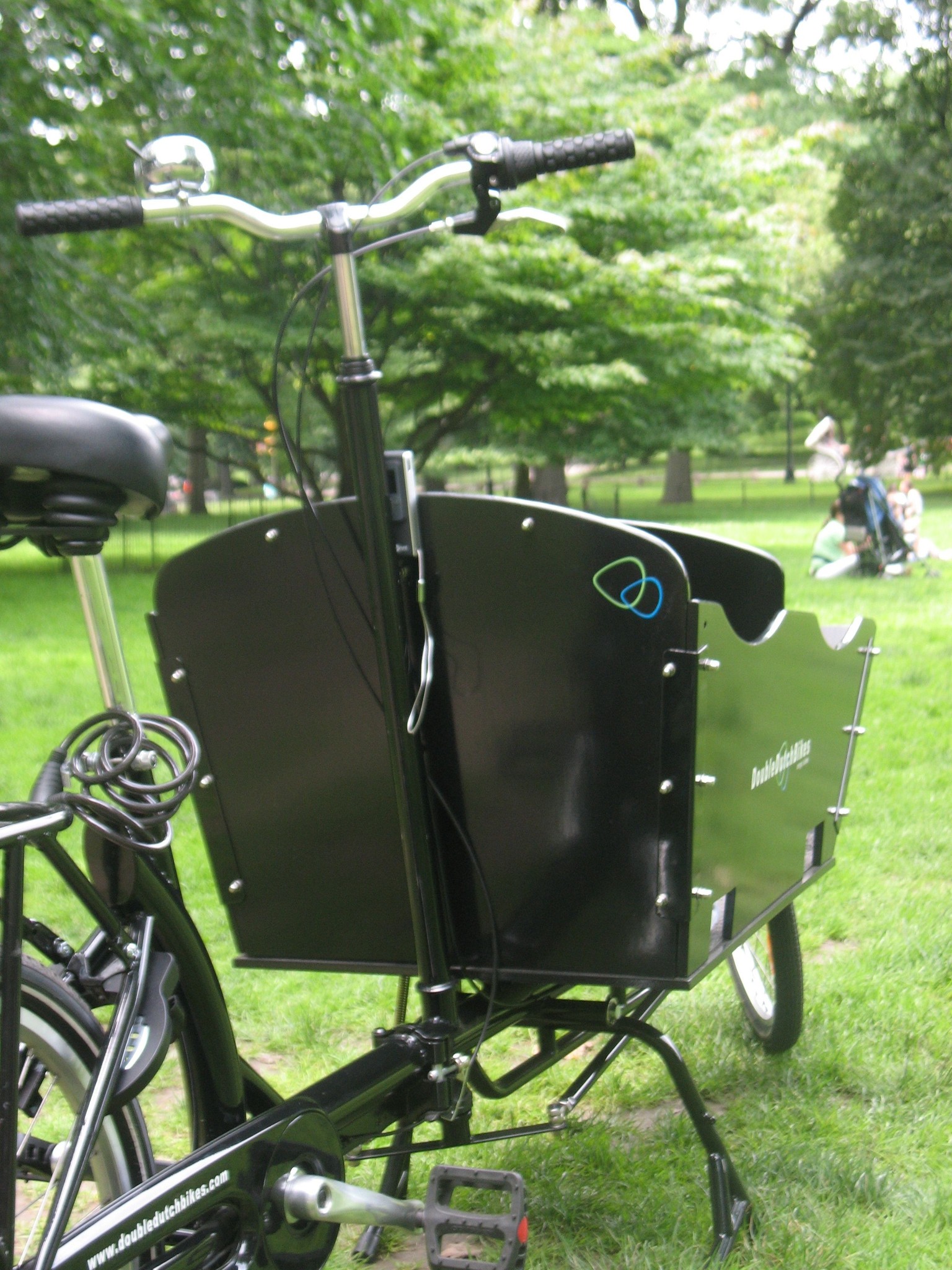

[0,135,890,1270]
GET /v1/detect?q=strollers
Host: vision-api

[830,467,922,582]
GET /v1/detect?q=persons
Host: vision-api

[809,500,871,581]
[888,478,924,561]
